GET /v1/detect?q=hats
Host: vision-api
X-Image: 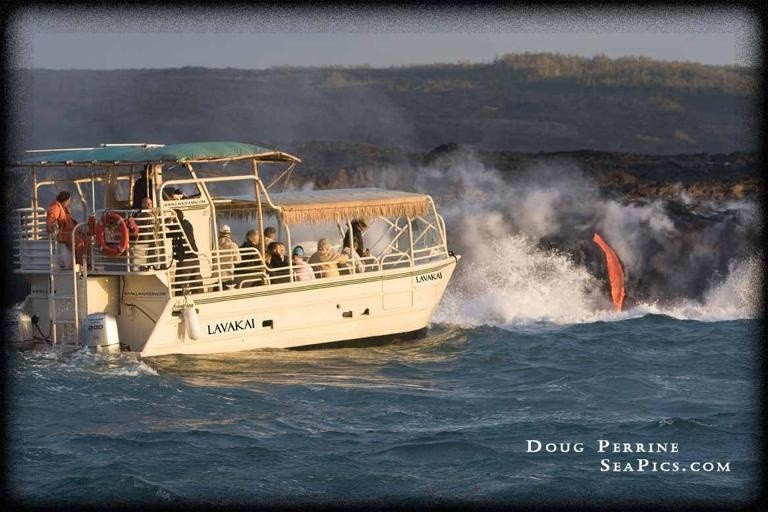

[291,245,305,256]
[219,223,231,234]
[55,191,71,203]
[358,216,370,226]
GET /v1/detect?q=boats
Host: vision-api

[5,140,463,358]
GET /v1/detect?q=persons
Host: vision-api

[46,165,371,295]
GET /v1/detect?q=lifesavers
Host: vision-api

[96,212,129,257]
